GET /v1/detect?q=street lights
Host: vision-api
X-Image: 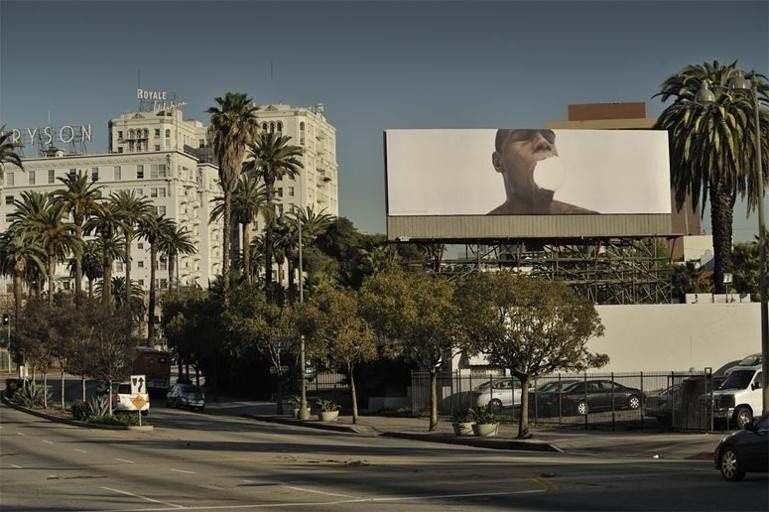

[4,312,16,374]
[296,208,311,420]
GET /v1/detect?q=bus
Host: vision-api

[268,351,317,382]
[95,345,171,391]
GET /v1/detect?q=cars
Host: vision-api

[166,382,207,409]
[645,353,764,431]
[471,377,646,416]
[170,357,206,386]
[714,412,769,482]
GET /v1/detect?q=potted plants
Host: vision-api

[287,395,341,422]
[452,407,500,437]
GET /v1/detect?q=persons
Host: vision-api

[489,129,597,215]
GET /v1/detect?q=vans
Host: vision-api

[95,378,151,416]
